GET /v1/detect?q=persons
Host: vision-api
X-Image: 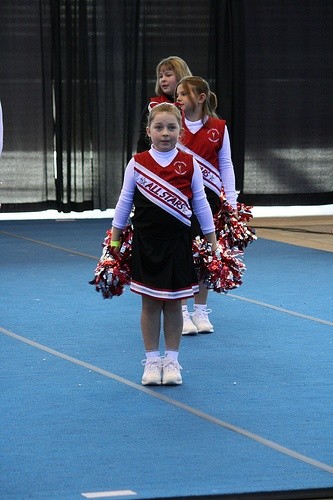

[135,56,238,335]
[111,105,218,386]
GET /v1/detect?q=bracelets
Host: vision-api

[110,241,120,248]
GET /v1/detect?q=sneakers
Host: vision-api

[190,309,214,333]
[141,359,162,385]
[162,358,183,385]
[181,311,198,334]
